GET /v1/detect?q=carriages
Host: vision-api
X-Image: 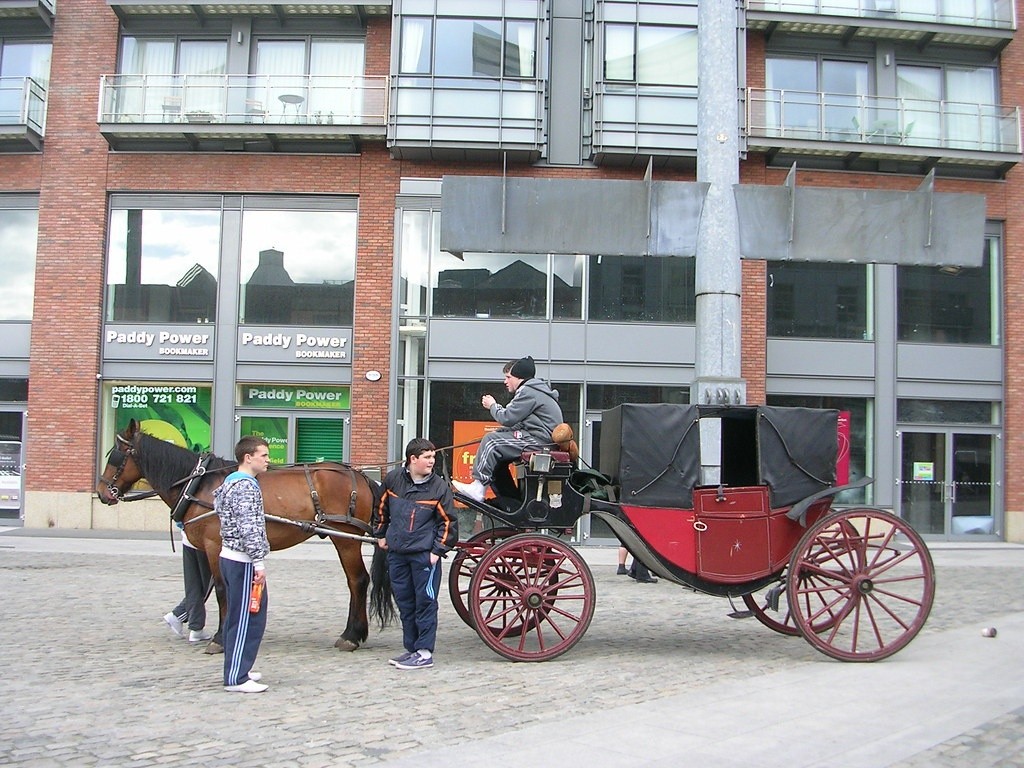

[94,401,936,663]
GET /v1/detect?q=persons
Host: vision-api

[163,528,215,641]
[451,356,563,513]
[212,437,270,692]
[373,438,459,670]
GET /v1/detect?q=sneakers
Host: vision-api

[247,671,261,682]
[388,653,412,664]
[452,479,490,502]
[485,497,521,511]
[223,679,269,693]
[163,612,186,639]
[395,652,433,669]
[189,626,215,642]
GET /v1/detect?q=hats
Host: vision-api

[509,356,537,380]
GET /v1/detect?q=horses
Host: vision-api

[97,418,399,655]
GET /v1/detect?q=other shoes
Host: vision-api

[617,568,630,575]
[636,577,658,583]
[628,569,637,579]
